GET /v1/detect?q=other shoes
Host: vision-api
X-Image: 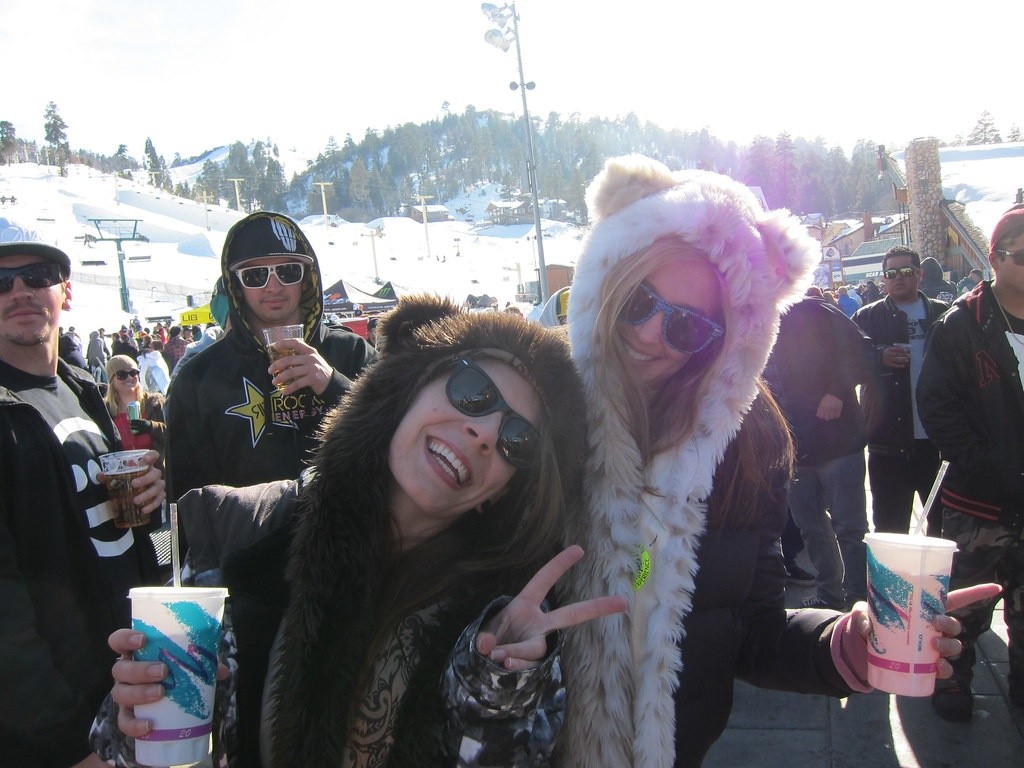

[931,687,974,722]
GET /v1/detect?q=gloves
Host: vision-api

[130,419,152,436]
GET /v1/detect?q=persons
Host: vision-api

[0,225,168,768]
[90,294,630,767]
[763,296,879,607]
[848,245,951,536]
[366,319,379,348]
[918,258,983,305]
[822,281,887,316]
[915,206,1024,726]
[323,308,362,325]
[165,211,379,564]
[466,294,522,318]
[561,155,1005,768]
[62,277,232,480]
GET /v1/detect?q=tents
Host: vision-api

[325,279,398,317]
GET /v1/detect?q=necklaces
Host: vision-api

[118,413,135,450]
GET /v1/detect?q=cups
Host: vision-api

[262,324,304,390]
[893,343,912,366]
[126,587,230,766]
[99,449,152,527]
[863,530,956,696]
[127,401,142,434]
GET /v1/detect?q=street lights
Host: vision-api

[481,0,552,302]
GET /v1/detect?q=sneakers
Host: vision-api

[844,591,866,603]
[799,595,834,609]
[784,560,816,586]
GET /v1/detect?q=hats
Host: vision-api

[989,204,1024,255]
[971,269,984,280]
[0,217,71,281]
[226,217,314,272]
[557,290,569,317]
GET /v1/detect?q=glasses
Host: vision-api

[235,262,305,289]
[110,369,139,383]
[445,358,542,471]
[618,284,725,355]
[884,266,914,279]
[997,250,1024,265]
[0,261,66,294]
